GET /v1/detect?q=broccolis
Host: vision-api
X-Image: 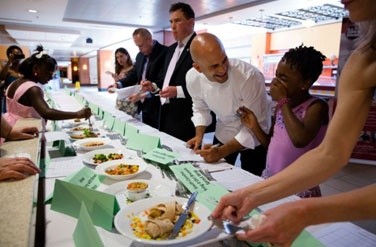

[95,154,106,161]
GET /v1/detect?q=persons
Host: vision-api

[0,115,42,181]
[210,0,376,247]
[236,44,329,197]
[0,46,24,89]
[185,33,272,163]
[0,45,91,145]
[112,48,138,118]
[107,28,169,129]
[140,2,198,142]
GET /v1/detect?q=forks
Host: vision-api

[211,216,249,236]
[88,117,93,132]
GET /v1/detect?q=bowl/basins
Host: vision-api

[113,196,213,246]
[75,137,111,151]
[83,149,138,165]
[95,159,147,181]
[125,181,150,201]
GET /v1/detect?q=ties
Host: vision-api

[140,56,150,104]
[160,41,183,106]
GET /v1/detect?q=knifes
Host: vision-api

[169,192,199,240]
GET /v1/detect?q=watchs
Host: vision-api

[276,97,291,108]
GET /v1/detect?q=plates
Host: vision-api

[65,118,110,140]
[103,179,173,213]
